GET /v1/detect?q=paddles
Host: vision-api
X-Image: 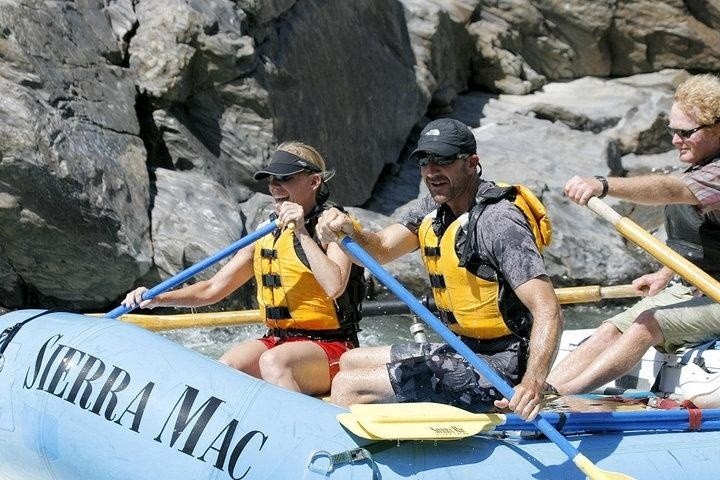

[336,402,718,442]
[334,229,632,479]
[337,399,719,441]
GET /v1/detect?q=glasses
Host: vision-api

[666,123,706,139]
[415,153,469,167]
[274,174,293,182]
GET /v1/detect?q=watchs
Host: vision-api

[594,174,608,196]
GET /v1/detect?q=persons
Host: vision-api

[118,139,364,395]
[313,119,563,423]
[541,73,720,395]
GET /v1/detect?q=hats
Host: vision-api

[254,149,323,181]
[408,117,477,160]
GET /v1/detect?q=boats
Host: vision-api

[0,307,720,480]
